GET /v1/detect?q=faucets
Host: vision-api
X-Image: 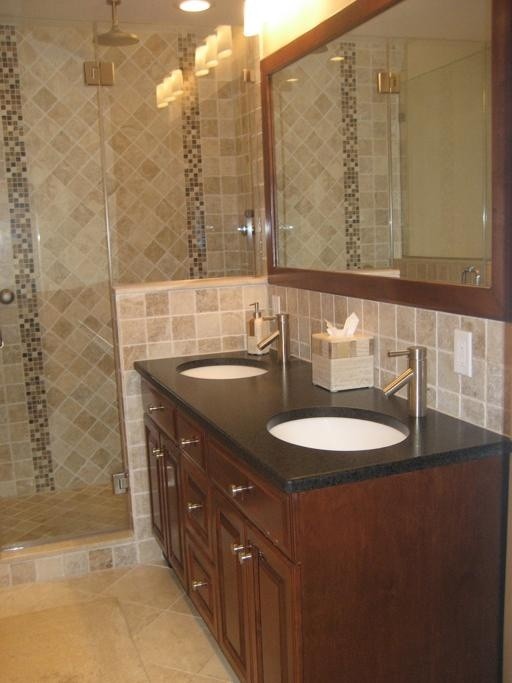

[257,314,290,361]
[383,346,427,419]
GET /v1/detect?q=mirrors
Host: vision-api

[260,0,512,323]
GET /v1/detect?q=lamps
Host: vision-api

[156,23,233,109]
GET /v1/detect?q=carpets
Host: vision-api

[1,593,151,683]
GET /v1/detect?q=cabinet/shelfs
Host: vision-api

[139,372,510,682]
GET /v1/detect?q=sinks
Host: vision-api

[266,406,410,452]
[176,358,269,380]
